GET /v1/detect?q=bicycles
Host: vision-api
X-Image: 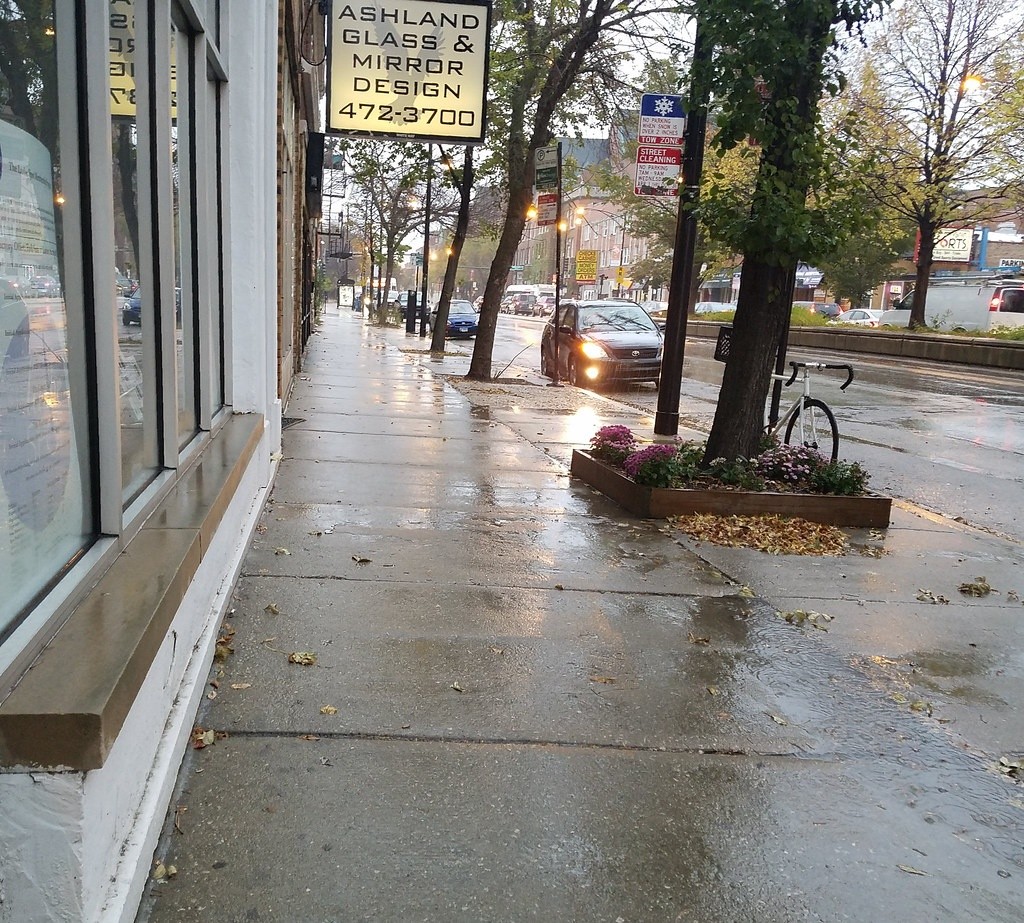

[761,360,855,463]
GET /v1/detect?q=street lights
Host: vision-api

[575,206,627,296]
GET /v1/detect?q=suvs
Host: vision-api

[540,296,666,392]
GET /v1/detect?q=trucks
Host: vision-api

[878,278,1024,329]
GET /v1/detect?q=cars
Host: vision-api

[531,296,556,317]
[792,300,843,322]
[500,296,513,313]
[826,309,884,328]
[374,291,399,316]
[430,299,479,339]
[394,291,431,324]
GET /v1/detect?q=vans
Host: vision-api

[510,294,537,315]
